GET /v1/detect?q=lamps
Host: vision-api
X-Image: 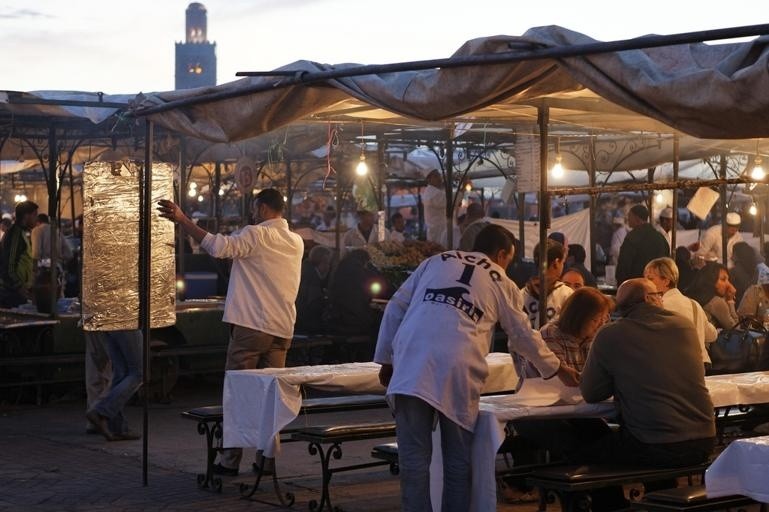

[752,140,765,181]
[357,120,369,176]
[552,136,564,180]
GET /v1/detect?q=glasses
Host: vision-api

[648,291,663,298]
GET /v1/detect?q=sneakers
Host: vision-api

[252,462,272,476]
[214,462,239,476]
[85,408,141,441]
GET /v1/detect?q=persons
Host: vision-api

[507,285,615,491]
[374,217,583,512]
[83,323,144,443]
[551,277,717,512]
[2,162,768,386]
[155,187,305,477]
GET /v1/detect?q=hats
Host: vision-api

[660,208,673,219]
[726,212,741,225]
[423,167,438,179]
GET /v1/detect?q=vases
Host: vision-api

[33,271,60,313]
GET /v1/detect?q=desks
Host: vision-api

[0,306,60,337]
[177,298,225,316]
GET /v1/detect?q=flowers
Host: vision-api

[37,257,62,278]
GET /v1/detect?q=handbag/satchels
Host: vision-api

[709,316,769,374]
